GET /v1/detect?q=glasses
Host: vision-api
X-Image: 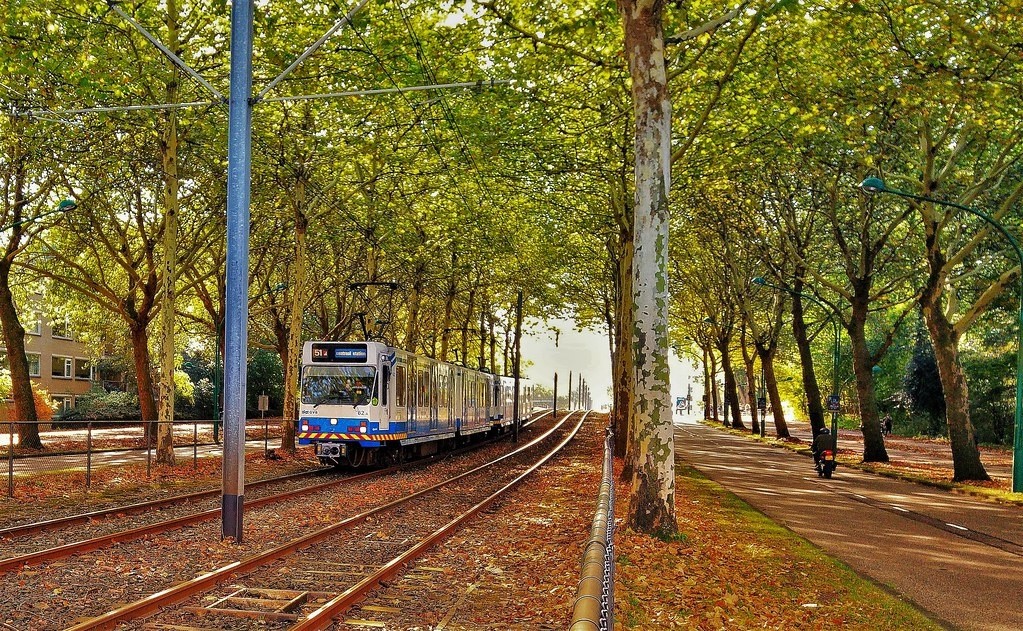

[356,381,363,382]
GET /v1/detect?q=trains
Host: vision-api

[293,337,534,468]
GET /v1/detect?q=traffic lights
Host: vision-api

[686,394,690,401]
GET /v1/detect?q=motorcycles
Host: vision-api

[811,444,834,475]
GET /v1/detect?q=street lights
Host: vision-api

[671,334,724,420]
[862,179,1022,493]
[752,277,882,440]
[706,316,794,436]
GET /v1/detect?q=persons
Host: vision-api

[810,428,836,471]
[858,411,893,440]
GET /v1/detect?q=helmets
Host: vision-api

[820,428,830,434]
